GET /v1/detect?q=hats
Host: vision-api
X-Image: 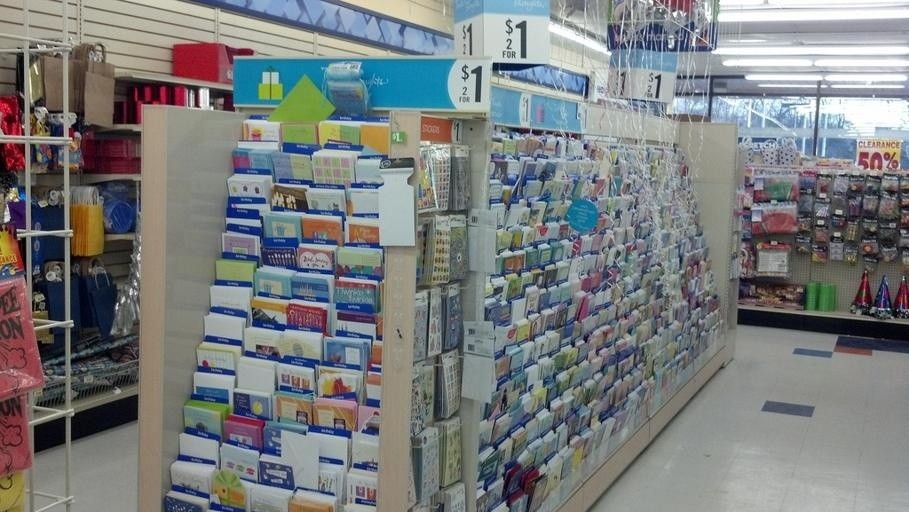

[849,268,909,319]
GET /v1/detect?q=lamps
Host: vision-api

[551,6,909,90]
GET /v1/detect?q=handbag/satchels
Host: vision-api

[47,185,117,339]
[43,43,115,129]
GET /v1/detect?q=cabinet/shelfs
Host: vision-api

[0,2,233,512]
